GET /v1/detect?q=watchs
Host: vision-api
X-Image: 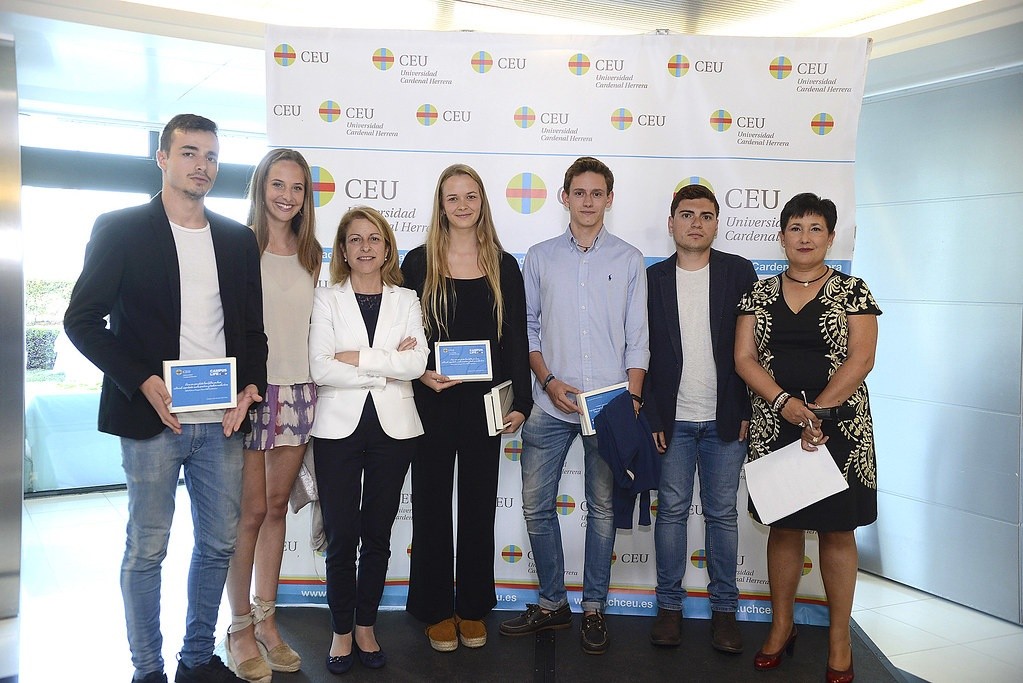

[630,395,645,406]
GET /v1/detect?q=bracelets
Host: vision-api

[812,402,822,409]
[771,391,793,414]
[541,373,556,391]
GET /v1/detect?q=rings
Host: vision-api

[799,422,805,428]
[812,437,818,443]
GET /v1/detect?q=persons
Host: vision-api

[308,205,430,676]
[63,114,269,683]
[499,157,652,654]
[400,164,534,652]
[734,193,884,683]
[223,149,323,683]
[646,184,759,654]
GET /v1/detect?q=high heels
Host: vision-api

[250,613,303,672]
[753,619,798,670]
[825,645,855,683]
[223,624,272,683]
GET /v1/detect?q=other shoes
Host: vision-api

[175,654,252,683]
[131,673,170,683]
[455,616,487,648]
[425,617,459,652]
[351,633,385,669]
[325,632,355,676]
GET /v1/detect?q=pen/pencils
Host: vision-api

[801,391,812,430]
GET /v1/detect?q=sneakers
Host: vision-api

[649,607,684,644]
[709,609,745,652]
[499,603,575,635]
[579,609,609,654]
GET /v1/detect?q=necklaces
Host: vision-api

[578,244,590,252]
[785,265,829,287]
